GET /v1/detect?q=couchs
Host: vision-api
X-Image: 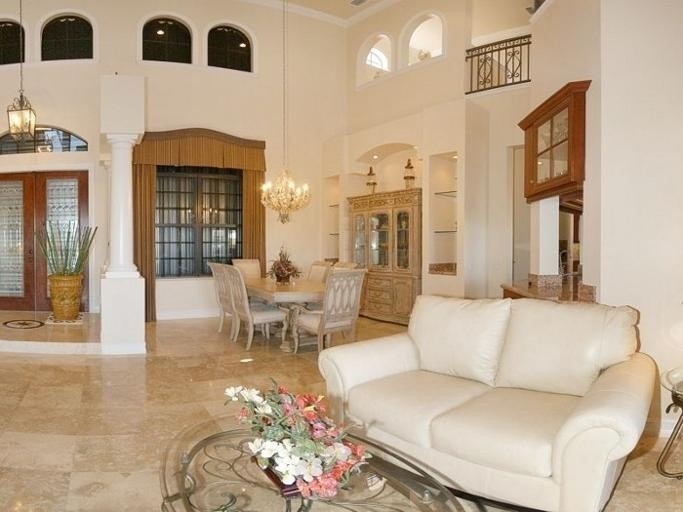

[318,293,661,512]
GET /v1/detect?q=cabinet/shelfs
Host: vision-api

[347,188,419,327]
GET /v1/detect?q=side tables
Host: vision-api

[657,364,683,480]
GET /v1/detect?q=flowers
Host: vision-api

[228,380,372,503]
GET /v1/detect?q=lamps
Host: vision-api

[6,1,36,143]
[259,1,311,224]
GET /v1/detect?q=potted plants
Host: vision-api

[34,219,98,323]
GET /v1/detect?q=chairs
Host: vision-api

[226,264,289,352]
[307,262,327,287]
[291,269,366,356]
[232,258,261,282]
[206,260,267,339]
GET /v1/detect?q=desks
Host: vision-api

[243,278,327,304]
